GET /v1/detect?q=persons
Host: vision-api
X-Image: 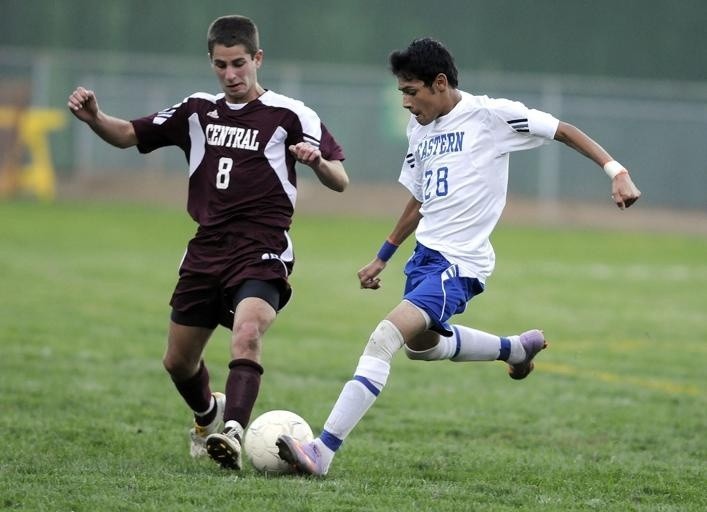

[276,35,641,476]
[65,13,348,474]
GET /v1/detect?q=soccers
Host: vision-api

[244,410,316,478]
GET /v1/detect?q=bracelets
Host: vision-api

[602,160,628,181]
[376,239,399,262]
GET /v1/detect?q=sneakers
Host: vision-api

[204,433,242,470]
[275,434,328,477]
[506,328,548,380]
[188,390,226,458]
